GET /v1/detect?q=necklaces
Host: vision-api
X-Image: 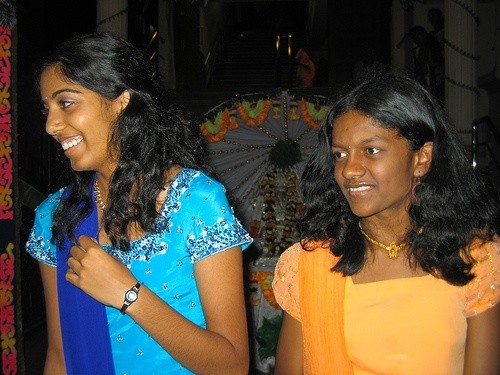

[93,179,137,225]
[357,219,428,259]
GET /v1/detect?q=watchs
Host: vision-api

[120,279,144,315]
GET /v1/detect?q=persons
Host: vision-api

[296,50,327,106]
[24,31,255,375]
[272,75,500,374]
